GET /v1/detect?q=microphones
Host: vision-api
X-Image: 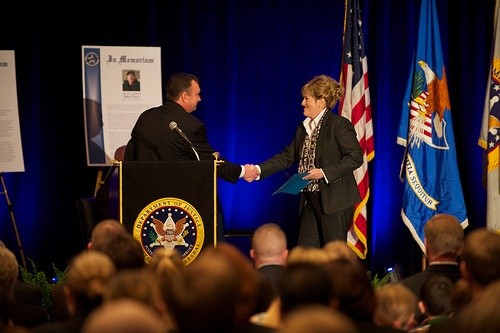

[168,121,193,147]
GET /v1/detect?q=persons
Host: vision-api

[245,74,364,246]
[122,70,140,91]
[0,214,499,333]
[124,73,260,185]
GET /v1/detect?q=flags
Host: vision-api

[336,0,377,261]
[394,0,470,274]
[478,0,499,233]
